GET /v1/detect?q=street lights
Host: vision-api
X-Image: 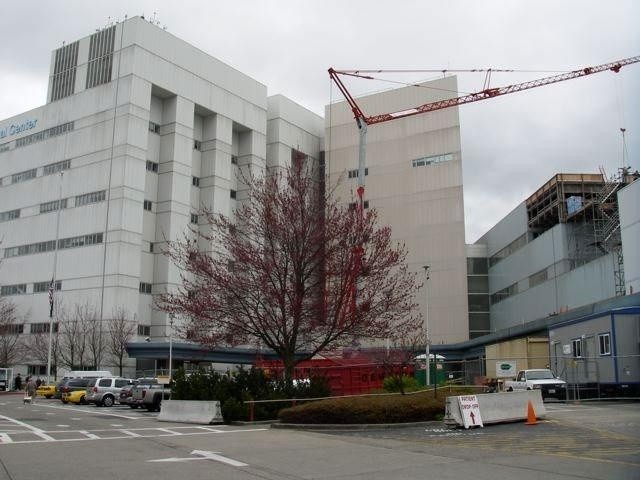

[167,309,175,383]
[420,261,431,386]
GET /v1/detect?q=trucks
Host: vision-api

[60,370,113,379]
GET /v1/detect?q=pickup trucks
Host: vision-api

[504,368,566,400]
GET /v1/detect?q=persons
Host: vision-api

[41,379,46,386]
[25,373,32,383]
[15,372,21,390]
[35,375,41,387]
[25,377,38,406]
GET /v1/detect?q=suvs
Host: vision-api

[36,377,173,415]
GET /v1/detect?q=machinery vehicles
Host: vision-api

[249,54,640,397]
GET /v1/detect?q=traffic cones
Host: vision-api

[525,399,539,426]
